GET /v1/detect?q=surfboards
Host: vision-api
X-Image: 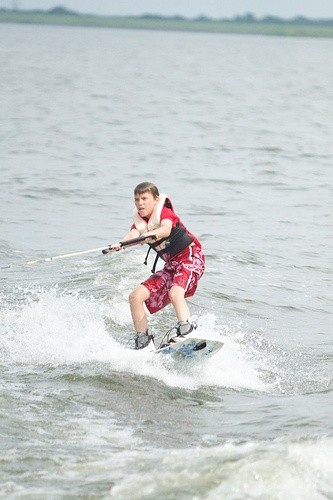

[150,337,225,366]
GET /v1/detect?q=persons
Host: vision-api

[103,182,205,350]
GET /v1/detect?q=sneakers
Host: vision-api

[126,330,154,349]
[158,320,198,349]
